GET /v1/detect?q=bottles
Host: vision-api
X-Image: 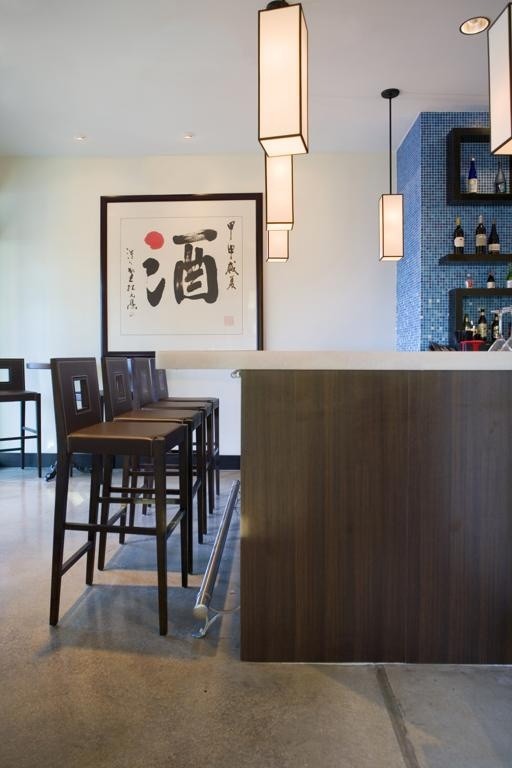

[494,160,506,193]
[465,273,473,289]
[453,218,465,254]
[467,159,478,194]
[487,220,500,255]
[463,309,499,343]
[505,264,512,289]
[474,215,487,255]
[486,274,495,288]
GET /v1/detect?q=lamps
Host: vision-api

[376,87,405,262]
[485,1,511,159]
[250,0,311,264]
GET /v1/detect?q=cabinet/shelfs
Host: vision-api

[431,126,511,351]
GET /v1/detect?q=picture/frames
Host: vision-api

[99,194,264,359]
